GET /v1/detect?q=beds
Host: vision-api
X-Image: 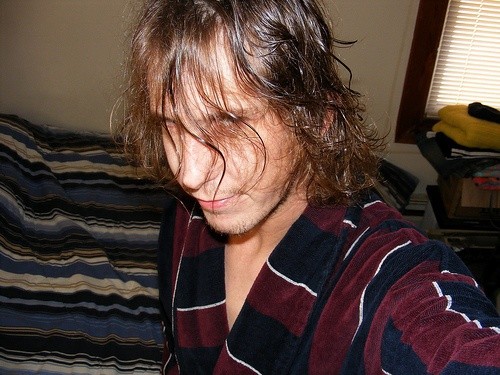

[0,113,174,375]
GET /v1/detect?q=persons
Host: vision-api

[109,0,500,375]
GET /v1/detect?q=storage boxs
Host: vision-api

[437,172,500,220]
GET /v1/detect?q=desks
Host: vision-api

[419,185,500,240]
